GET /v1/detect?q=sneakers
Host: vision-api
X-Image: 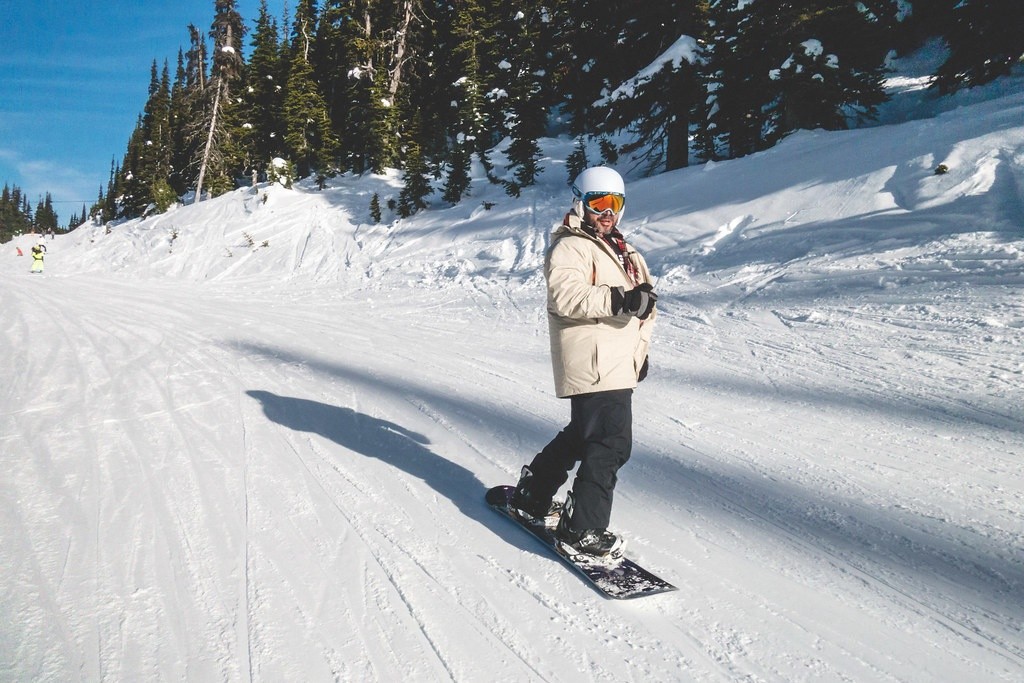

[507,464,627,559]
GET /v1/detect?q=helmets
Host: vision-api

[572,167,625,228]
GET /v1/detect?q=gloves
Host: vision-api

[638,355,649,382]
[623,282,658,319]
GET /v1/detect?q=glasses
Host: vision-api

[572,183,625,215]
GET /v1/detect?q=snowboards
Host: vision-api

[485,484,679,599]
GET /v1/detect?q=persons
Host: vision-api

[31,243,46,274]
[246,388,482,510]
[511,166,657,557]
[29,225,55,239]
[15,247,23,256]
